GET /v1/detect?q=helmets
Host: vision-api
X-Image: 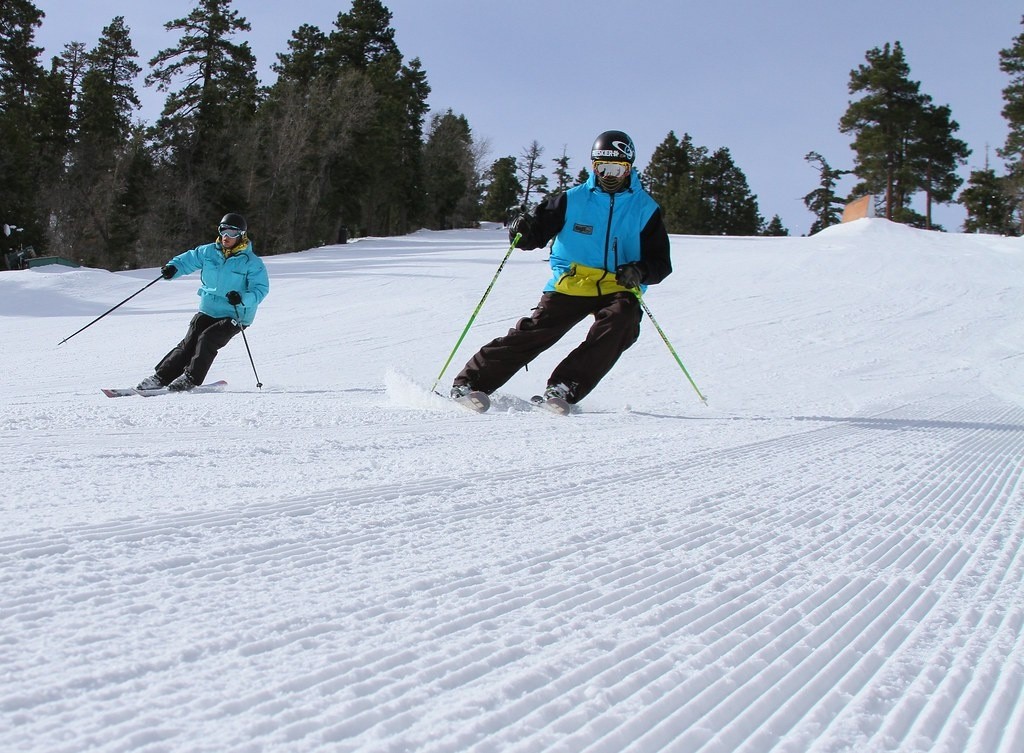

[591,130,635,164]
[220,213,247,232]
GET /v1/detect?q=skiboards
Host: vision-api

[532,398,572,417]
[100,380,230,398]
[452,391,491,415]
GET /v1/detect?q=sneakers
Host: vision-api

[450,386,473,399]
[544,385,567,402]
[136,376,163,390]
[165,373,196,392]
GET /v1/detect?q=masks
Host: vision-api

[597,177,625,193]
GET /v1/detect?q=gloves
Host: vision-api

[509,212,536,239]
[225,290,241,305]
[615,261,647,290]
[161,265,178,279]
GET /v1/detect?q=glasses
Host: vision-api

[592,161,631,179]
[218,224,246,239]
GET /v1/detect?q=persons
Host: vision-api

[450,130,672,401]
[136,213,269,391]
